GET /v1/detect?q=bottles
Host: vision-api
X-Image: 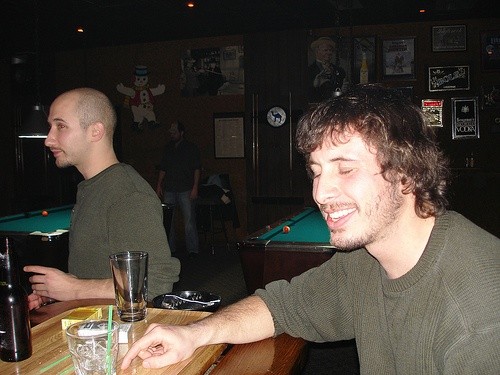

[0,236,33,362]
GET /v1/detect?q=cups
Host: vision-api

[109,250,149,322]
[65,318,120,375]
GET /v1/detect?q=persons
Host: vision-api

[120,82,500,375]
[184,58,204,97]
[24,87,181,311]
[156,120,202,258]
[303,36,346,96]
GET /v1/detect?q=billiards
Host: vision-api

[282,226,290,234]
[42,210,48,216]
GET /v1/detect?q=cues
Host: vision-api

[251,92,259,197]
[13,103,25,194]
[289,91,292,192]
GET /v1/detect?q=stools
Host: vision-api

[198,174,228,256]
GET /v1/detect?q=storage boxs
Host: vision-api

[61,307,133,343]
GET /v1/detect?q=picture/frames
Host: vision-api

[348,25,500,140]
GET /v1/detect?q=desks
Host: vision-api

[0,299,308,375]
[236,205,338,295]
[0,202,173,295]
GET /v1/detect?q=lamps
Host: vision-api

[17,8,50,139]
